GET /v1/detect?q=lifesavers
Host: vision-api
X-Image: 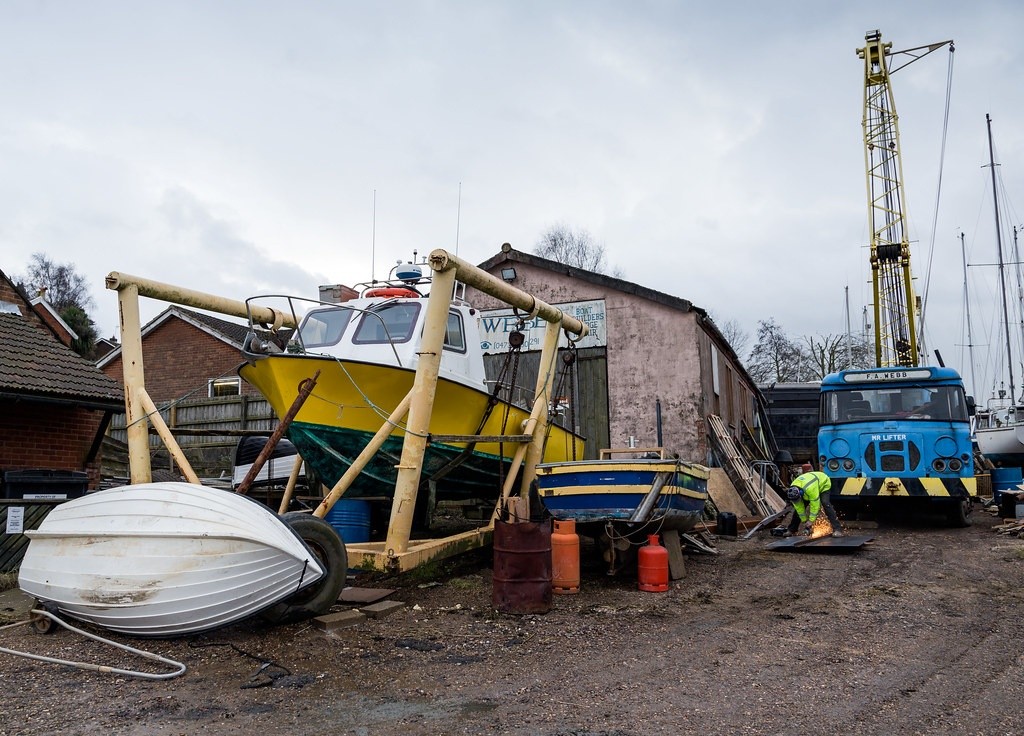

[364,287,419,297]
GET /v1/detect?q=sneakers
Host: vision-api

[783,531,792,537]
[832,531,844,537]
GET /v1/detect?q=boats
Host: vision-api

[17,481,325,638]
[239,181,587,512]
[536,448,711,536]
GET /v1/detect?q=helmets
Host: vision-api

[787,487,799,501]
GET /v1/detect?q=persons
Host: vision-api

[783,472,844,538]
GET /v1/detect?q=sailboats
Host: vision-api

[961,112,1024,457]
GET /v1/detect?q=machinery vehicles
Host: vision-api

[813,29,979,523]
